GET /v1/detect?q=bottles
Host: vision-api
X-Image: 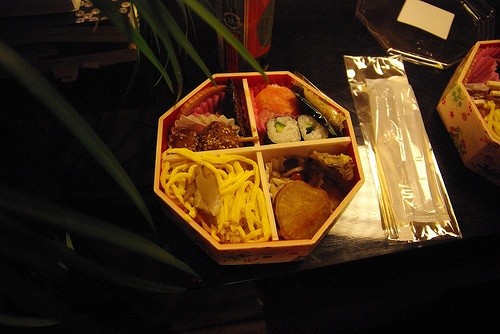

[215,0,274,74]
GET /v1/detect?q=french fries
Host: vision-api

[160,149,270,243]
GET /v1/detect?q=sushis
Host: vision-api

[297,114,329,141]
[263,115,302,144]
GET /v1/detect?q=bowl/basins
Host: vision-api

[153,71,365,265]
[436,39,500,185]
